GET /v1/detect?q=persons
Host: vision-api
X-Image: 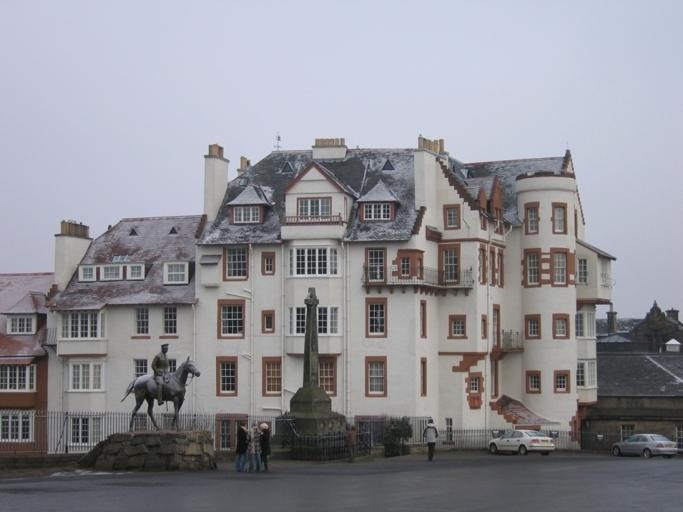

[422,417,438,461]
[234,420,247,473]
[246,425,260,473]
[344,423,357,464]
[259,422,271,474]
[149,343,171,406]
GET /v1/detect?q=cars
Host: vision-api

[611,433,679,460]
[487,429,556,456]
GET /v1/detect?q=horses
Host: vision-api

[119,355,201,432]
